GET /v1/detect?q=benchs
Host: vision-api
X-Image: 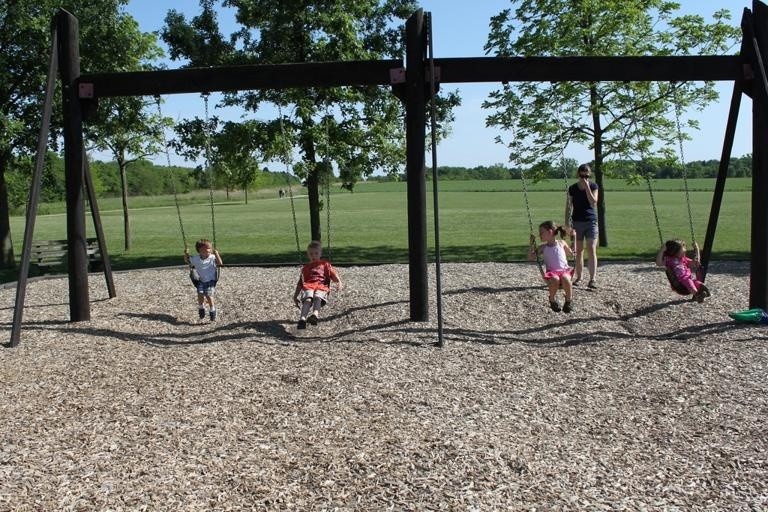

[30,237,99,274]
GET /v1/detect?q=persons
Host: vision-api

[529,220,577,314]
[293,240,343,329]
[566,164,599,289]
[655,236,710,303]
[185,238,222,322]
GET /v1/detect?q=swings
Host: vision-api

[501,76,573,285]
[156,87,219,288]
[623,73,702,294]
[275,85,331,308]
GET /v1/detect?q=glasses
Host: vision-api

[579,174,589,179]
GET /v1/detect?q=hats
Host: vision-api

[666,240,686,257]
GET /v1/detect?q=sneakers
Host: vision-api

[573,278,583,286]
[587,279,596,288]
[199,308,205,319]
[550,300,574,313]
[210,310,217,322]
[694,284,711,303]
[298,314,318,329]
